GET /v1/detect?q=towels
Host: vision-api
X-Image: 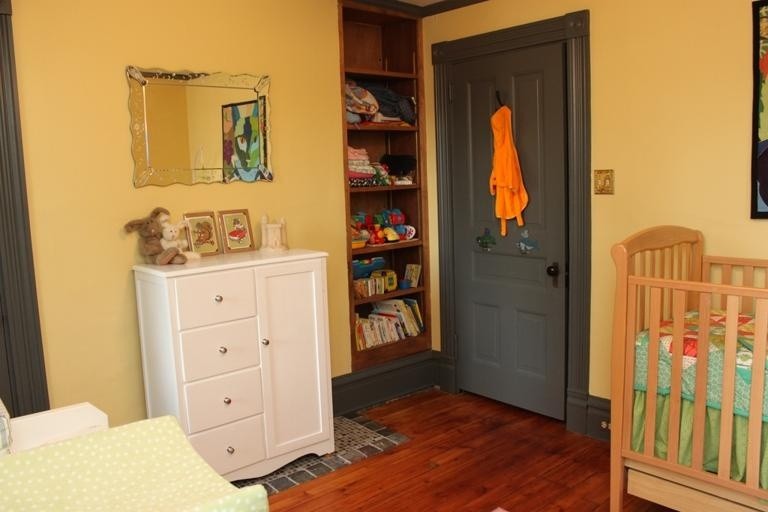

[488,106,530,237]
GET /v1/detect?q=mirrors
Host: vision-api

[123,65,275,188]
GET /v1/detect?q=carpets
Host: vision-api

[230,410,410,498]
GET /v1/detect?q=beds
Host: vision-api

[610,226,768,512]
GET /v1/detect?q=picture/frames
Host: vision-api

[751,0,768,220]
[221,96,268,183]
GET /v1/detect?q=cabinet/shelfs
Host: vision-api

[336,2,432,374]
[132,248,336,484]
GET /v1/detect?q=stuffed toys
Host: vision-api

[160,214,201,265]
[125,208,186,266]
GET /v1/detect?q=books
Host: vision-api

[351,252,426,351]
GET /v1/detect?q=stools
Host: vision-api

[0,396,271,512]
